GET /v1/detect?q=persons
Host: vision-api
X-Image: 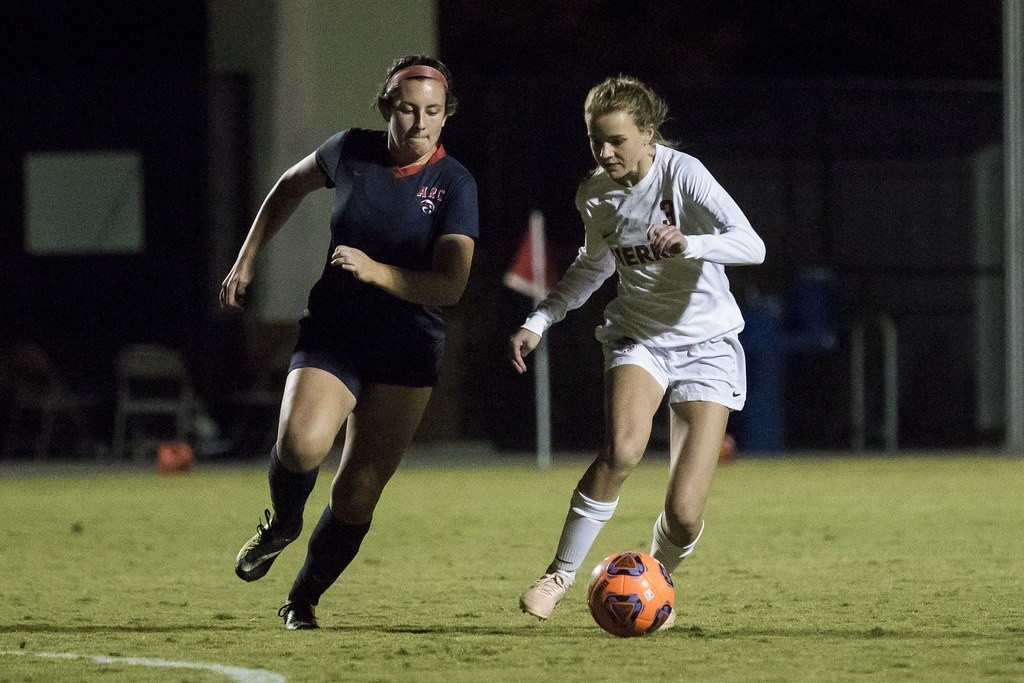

[508,77,766,631]
[219,54,479,631]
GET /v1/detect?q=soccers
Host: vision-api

[587,552,675,638]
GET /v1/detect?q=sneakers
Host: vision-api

[233,510,304,583]
[659,603,678,632]
[519,566,577,621]
[278,584,321,633]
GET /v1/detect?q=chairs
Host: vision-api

[117,345,188,466]
